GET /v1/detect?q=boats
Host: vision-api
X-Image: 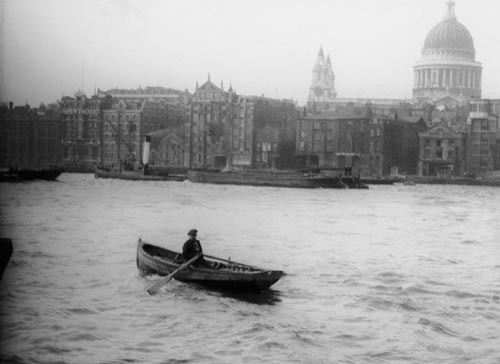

[136,239,284,293]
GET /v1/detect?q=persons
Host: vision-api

[182,229,206,267]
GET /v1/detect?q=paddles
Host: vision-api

[147,254,201,294]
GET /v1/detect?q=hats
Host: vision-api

[187,229,197,235]
